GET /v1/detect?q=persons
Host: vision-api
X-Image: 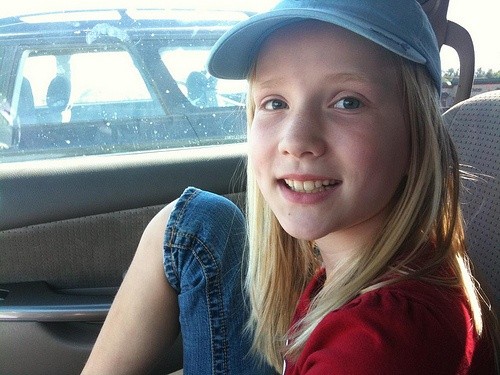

[78,0,500,375]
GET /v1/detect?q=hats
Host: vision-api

[207,0,441,95]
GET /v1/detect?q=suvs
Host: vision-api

[0,8,258,161]
